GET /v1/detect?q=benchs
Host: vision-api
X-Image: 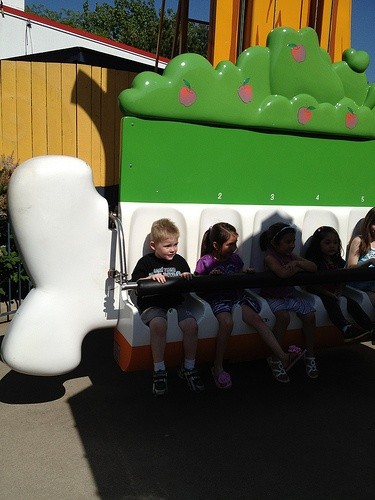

[0,27,375,374]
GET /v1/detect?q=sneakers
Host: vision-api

[151,368,169,398]
[178,364,206,393]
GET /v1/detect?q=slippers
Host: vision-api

[285,344,308,372]
[211,366,232,388]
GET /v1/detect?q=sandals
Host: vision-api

[304,353,318,378]
[267,355,290,382]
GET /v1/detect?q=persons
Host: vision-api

[347,207,375,300]
[193,222,307,390]
[259,223,319,382]
[303,226,375,342]
[130,219,206,394]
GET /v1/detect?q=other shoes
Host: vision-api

[343,326,370,342]
